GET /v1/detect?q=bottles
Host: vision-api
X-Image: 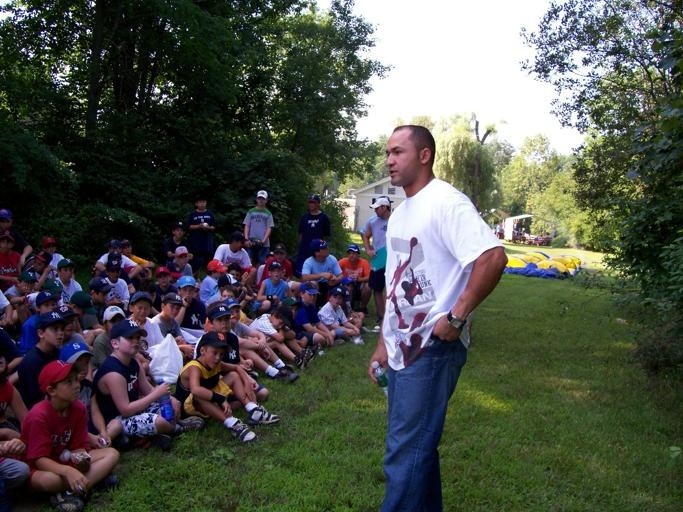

[371,361,389,399]
[157,379,175,422]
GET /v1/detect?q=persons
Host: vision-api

[353,126,509,510]
[361,196,396,330]
[1,189,372,511]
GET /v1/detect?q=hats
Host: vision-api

[207,260,240,320]
[231,230,248,241]
[331,277,354,300]
[0,209,15,242]
[71,291,98,314]
[108,239,130,269]
[37,359,75,392]
[346,245,359,252]
[310,239,328,249]
[89,266,197,340]
[256,190,268,199]
[36,305,79,329]
[266,243,288,257]
[175,246,188,257]
[283,283,319,306]
[201,332,228,348]
[174,221,186,230]
[368,197,391,209]
[18,237,74,306]
[269,263,284,274]
[59,340,94,364]
[308,195,320,204]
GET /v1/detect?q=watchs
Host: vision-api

[447,311,466,329]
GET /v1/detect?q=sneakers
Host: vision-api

[96,476,120,493]
[224,419,257,443]
[334,300,383,346]
[246,402,282,426]
[112,416,205,451]
[247,339,326,383]
[49,493,84,511]
